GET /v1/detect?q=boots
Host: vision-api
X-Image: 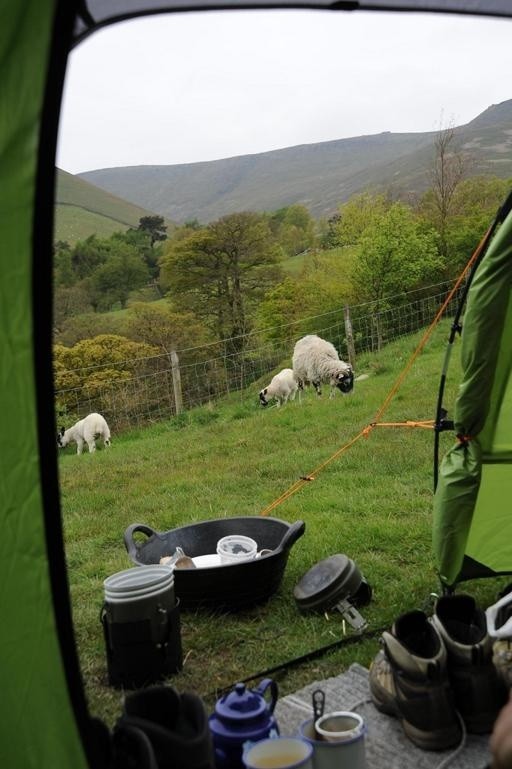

[368,594,501,749]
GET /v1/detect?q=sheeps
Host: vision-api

[56,412,111,453]
[259,368,298,408]
[292,334,355,399]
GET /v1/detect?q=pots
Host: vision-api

[296,554,370,633]
[123,509,306,607]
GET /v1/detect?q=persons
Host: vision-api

[488,684,512,769]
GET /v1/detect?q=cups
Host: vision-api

[239,713,367,766]
[215,533,256,562]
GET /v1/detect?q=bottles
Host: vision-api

[205,675,278,767]
